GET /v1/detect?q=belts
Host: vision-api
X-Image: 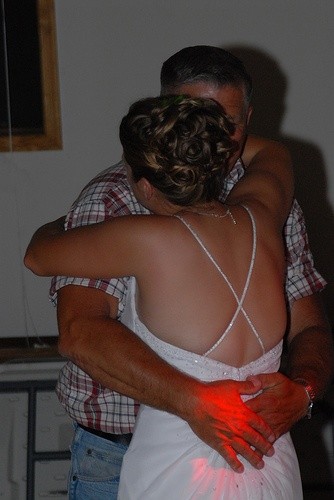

[76,423,132,446]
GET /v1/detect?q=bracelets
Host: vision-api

[291,376,316,420]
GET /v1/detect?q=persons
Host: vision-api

[23,96,303,500]
[47,45,334,500]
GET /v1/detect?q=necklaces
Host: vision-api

[196,204,237,225]
